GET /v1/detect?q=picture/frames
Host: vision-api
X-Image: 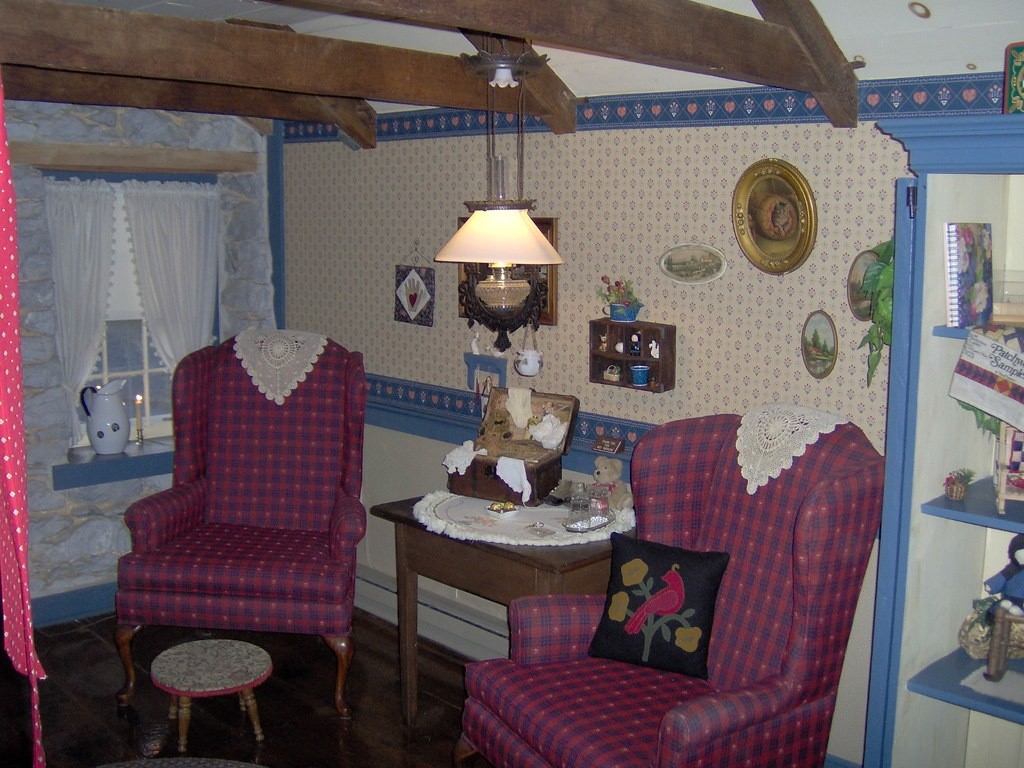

[660,242,727,286]
[1003,42,1024,113]
[458,216,559,325]
[801,310,836,379]
[733,158,818,275]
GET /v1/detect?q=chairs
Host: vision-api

[453,414,885,768]
[116,333,364,706]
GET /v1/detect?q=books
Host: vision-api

[942,222,994,329]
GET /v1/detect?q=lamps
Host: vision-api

[434,35,564,351]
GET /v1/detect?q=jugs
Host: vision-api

[80,379,131,455]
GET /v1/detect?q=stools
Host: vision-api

[151,638,274,753]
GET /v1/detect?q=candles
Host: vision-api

[135,395,143,430]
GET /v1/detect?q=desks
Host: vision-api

[369,491,615,725]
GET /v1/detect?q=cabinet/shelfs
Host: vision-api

[862,113,1024,768]
[589,317,677,394]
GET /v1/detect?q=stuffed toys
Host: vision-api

[984,534,1024,616]
[585,456,634,510]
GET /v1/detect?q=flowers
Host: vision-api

[596,276,640,309]
[946,469,975,486]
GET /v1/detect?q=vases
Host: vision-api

[609,303,644,323]
[945,485,965,501]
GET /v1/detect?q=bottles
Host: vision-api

[514,350,544,377]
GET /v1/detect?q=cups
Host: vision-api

[630,365,650,387]
[567,496,590,529]
[588,488,610,522]
[570,482,587,497]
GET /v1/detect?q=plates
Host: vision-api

[561,509,616,533]
[486,506,519,519]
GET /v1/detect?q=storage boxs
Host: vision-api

[448,384,580,507]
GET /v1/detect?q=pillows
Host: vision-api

[590,534,730,677]
